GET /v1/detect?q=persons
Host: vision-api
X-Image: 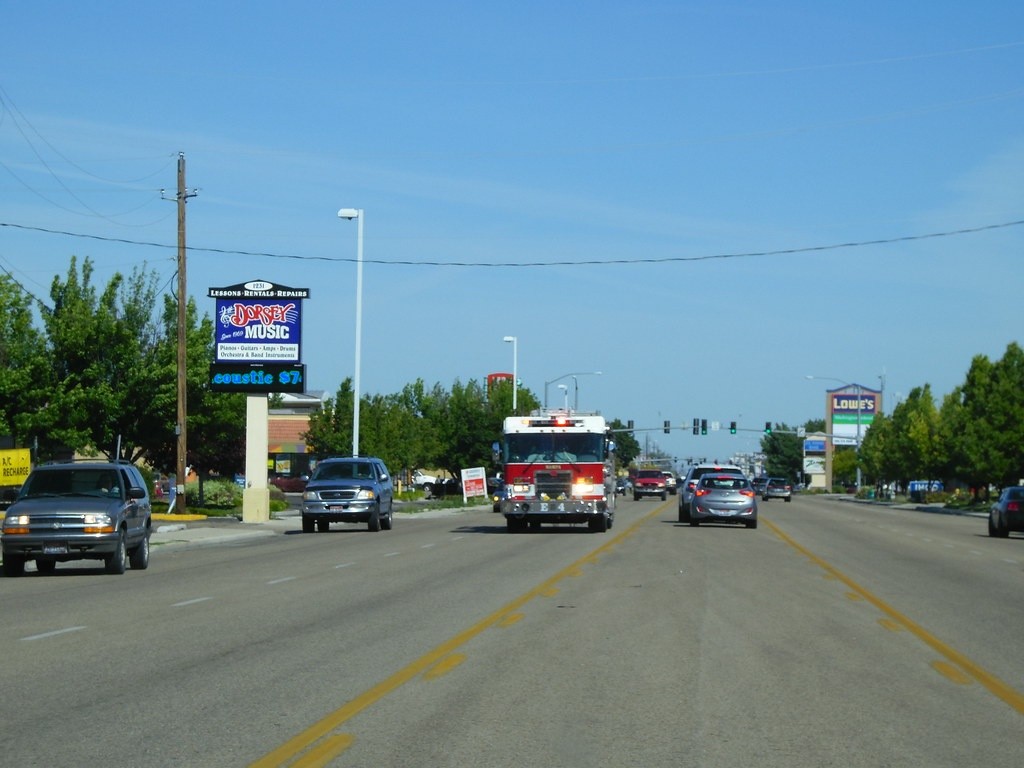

[99,475,120,497]
[435,475,441,484]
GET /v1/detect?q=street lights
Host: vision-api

[557,384,567,409]
[804,374,861,494]
[503,336,518,410]
[336,208,363,478]
[571,375,578,410]
[545,371,602,408]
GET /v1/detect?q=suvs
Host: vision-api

[302,454,393,533]
[630,469,669,501]
[678,462,745,523]
[412,469,437,491]
[751,476,769,495]
[0,458,152,577]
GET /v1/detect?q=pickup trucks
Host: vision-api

[661,471,677,495]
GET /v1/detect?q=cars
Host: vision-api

[493,478,504,513]
[616,478,633,496]
[762,478,792,502]
[689,472,758,529]
[988,486,1024,539]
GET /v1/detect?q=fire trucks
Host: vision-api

[493,410,616,533]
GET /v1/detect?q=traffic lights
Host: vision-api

[765,421,771,434]
[730,421,736,434]
[701,419,707,435]
